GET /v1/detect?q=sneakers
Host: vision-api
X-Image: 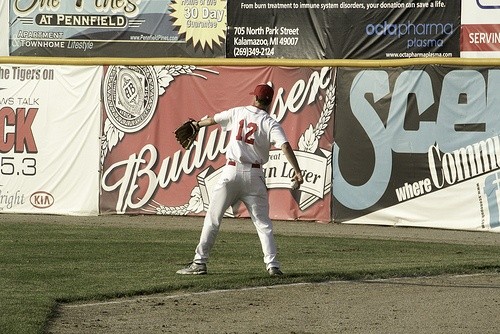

[176,262,207,275]
[269,268,283,275]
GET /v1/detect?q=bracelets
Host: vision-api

[197,121,201,128]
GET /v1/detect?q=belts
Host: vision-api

[228,162,260,168]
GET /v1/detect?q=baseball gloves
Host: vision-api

[174,118,200,150]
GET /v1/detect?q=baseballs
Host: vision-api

[290,180,299,189]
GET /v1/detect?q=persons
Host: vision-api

[176,84,305,276]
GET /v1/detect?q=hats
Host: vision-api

[249,85,274,102]
